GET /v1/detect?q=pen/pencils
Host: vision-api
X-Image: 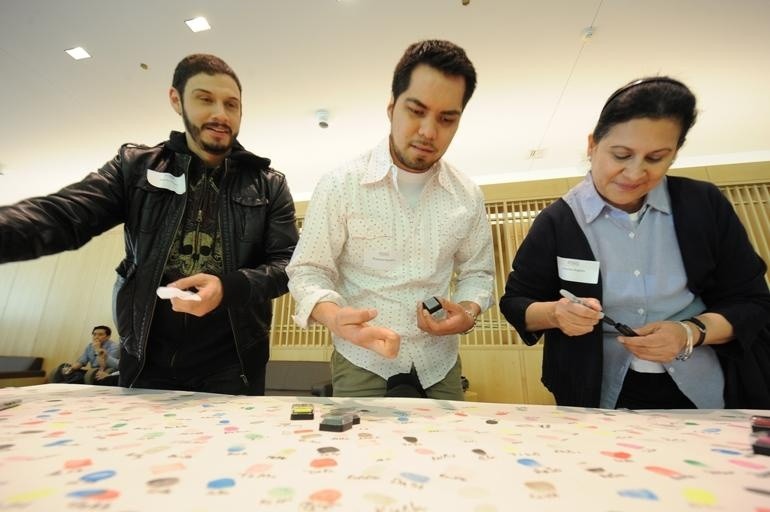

[559,289,617,326]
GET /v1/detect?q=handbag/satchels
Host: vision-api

[53,363,88,384]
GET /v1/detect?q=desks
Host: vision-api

[0,383,770,512]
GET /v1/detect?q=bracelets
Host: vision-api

[462,310,476,337]
[677,319,694,362]
[682,317,708,348]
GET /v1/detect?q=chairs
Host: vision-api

[263,360,333,397]
[0,356,48,389]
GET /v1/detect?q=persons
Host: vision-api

[91,349,121,386]
[1,55,300,395]
[53,325,120,382]
[285,39,497,403]
[498,73,768,408]
